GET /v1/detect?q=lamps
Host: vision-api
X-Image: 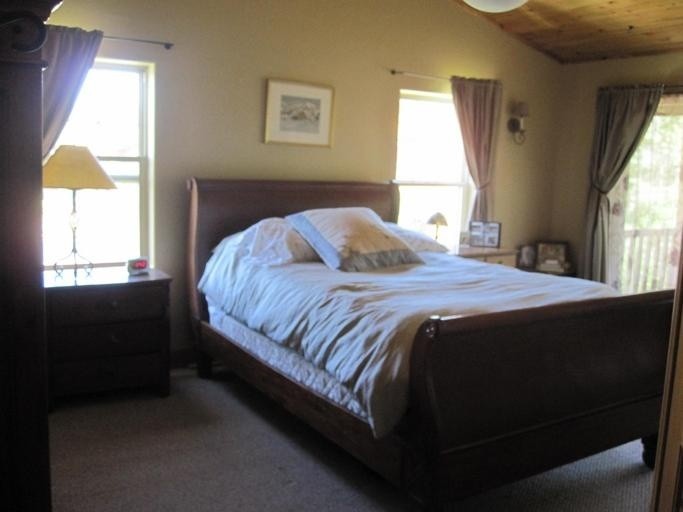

[507,100,530,145]
[426,212,448,241]
[42,145,118,276]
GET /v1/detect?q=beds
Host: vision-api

[182,176,676,512]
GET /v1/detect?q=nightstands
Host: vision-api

[42,265,173,399]
[457,246,520,267]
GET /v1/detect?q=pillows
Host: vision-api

[239,207,450,273]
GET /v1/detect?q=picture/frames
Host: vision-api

[262,77,336,149]
[517,241,569,273]
[469,220,502,249]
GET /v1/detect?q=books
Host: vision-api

[534,258,565,273]
